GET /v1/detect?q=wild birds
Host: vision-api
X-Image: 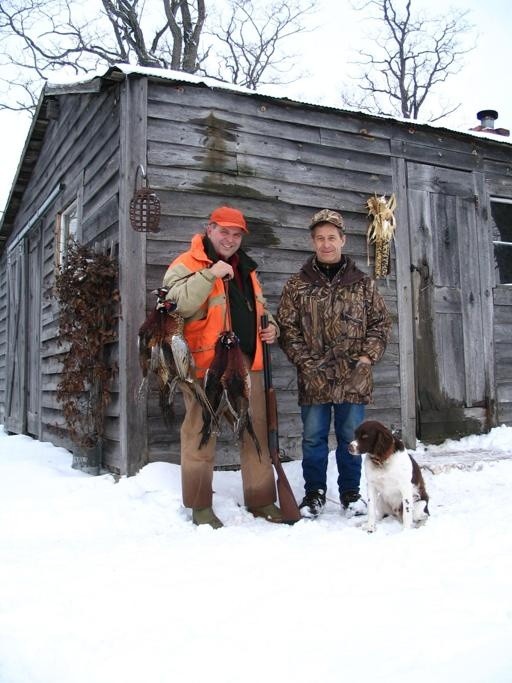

[137,285,222,431]
[210,332,262,465]
[197,331,229,449]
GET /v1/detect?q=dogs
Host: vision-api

[348,420,431,534]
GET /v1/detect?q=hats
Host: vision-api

[209,207,249,232]
[307,210,345,231]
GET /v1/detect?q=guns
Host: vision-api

[261,314,302,525]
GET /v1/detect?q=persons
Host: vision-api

[277,209,392,519]
[162,207,297,529]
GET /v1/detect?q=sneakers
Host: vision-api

[299,492,326,520]
[193,507,226,529]
[341,493,368,517]
[248,504,293,523]
